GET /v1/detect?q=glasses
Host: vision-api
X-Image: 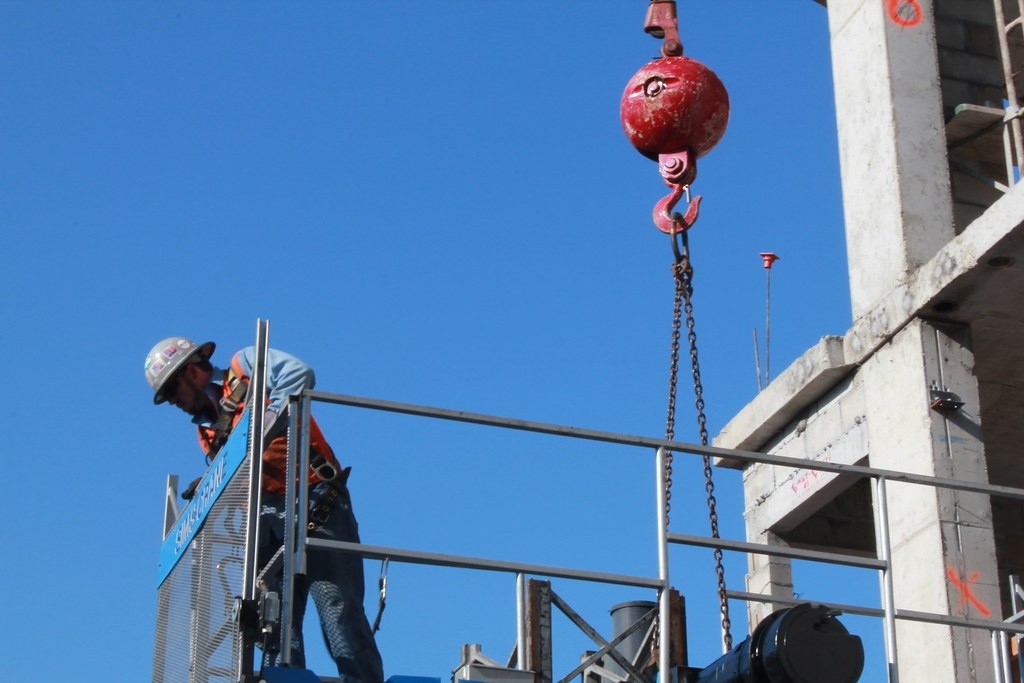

[162,365,186,400]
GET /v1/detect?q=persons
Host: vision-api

[142,337,383,683]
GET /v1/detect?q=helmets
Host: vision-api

[145,337,216,405]
